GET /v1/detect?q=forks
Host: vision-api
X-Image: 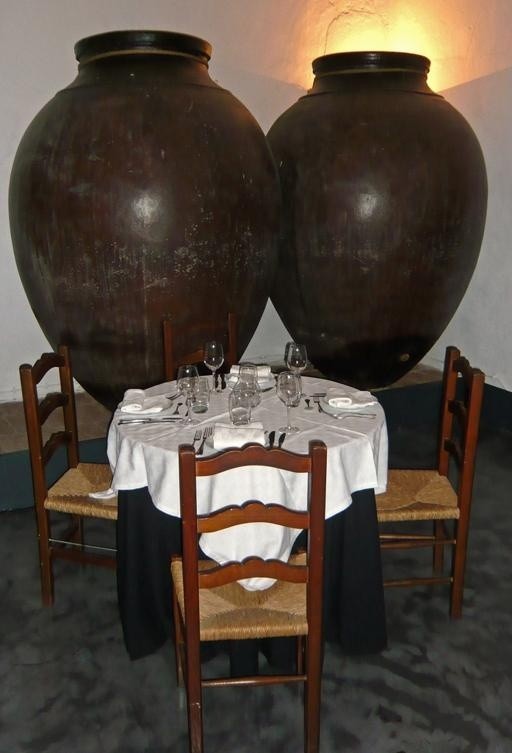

[312,394,322,414]
[190,428,202,446]
[194,426,213,456]
[317,403,378,422]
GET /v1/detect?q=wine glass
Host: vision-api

[286,342,309,398]
[203,340,225,395]
[276,371,304,435]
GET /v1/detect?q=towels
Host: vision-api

[229,365,271,383]
[121,388,165,415]
[213,423,265,451]
[327,387,378,408]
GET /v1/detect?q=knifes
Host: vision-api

[117,416,182,426]
[264,430,286,448]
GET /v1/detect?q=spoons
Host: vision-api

[172,402,184,415]
[304,398,315,410]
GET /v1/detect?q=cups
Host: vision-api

[189,377,211,413]
[177,363,201,398]
[227,362,264,426]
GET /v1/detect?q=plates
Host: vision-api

[226,372,276,392]
[204,428,268,451]
[323,392,378,412]
[117,397,172,416]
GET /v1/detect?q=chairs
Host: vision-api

[377,346,485,618]
[170,439,327,753]
[19,340,118,609]
